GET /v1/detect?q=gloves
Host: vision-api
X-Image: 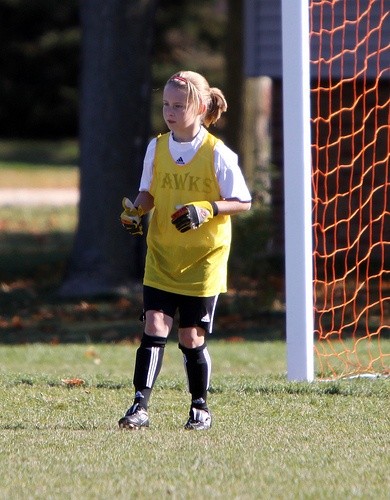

[170,201,218,233]
[121,197,143,235]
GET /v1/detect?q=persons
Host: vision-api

[116,68,253,433]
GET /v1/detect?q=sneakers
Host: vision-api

[184,407,213,430]
[118,402,149,429]
